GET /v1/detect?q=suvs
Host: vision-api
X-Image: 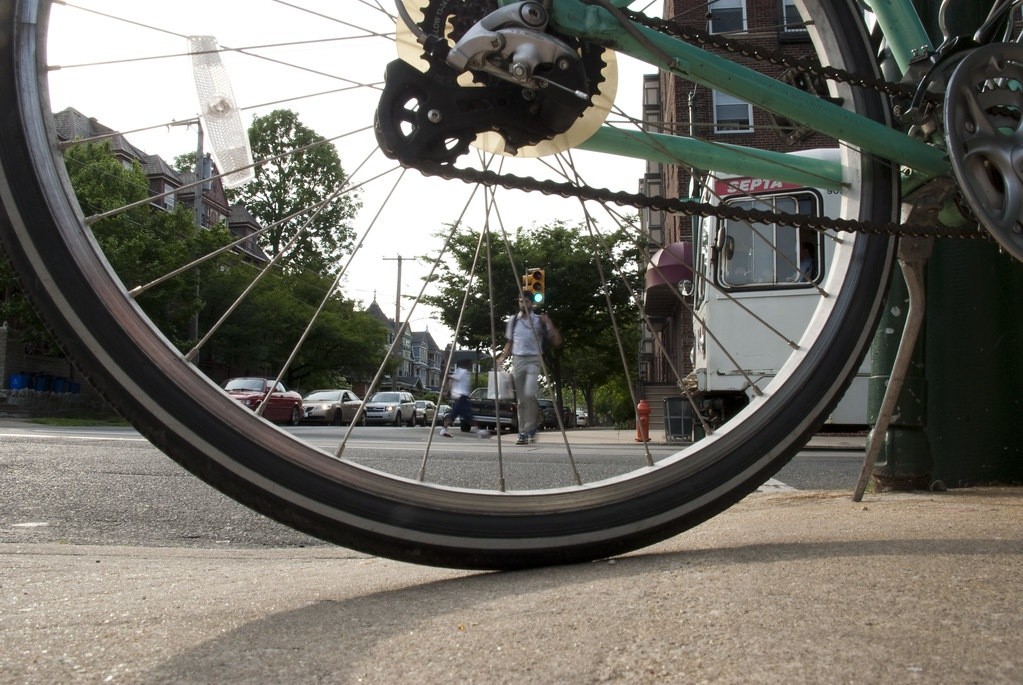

[364,390,418,428]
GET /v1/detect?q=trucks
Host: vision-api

[678,145,869,447]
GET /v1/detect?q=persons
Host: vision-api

[499,290,563,445]
[437,360,473,438]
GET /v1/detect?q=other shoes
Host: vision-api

[477,429,489,436]
[529,431,536,443]
[516,432,528,445]
[439,429,452,438]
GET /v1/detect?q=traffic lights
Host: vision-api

[521,271,532,309]
[530,268,545,304]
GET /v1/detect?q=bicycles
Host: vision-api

[0,1,1023,572]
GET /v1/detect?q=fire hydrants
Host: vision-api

[633,399,651,443]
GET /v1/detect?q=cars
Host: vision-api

[217,377,305,427]
[300,388,368,427]
[457,385,588,433]
[414,399,438,427]
[436,405,453,428]
